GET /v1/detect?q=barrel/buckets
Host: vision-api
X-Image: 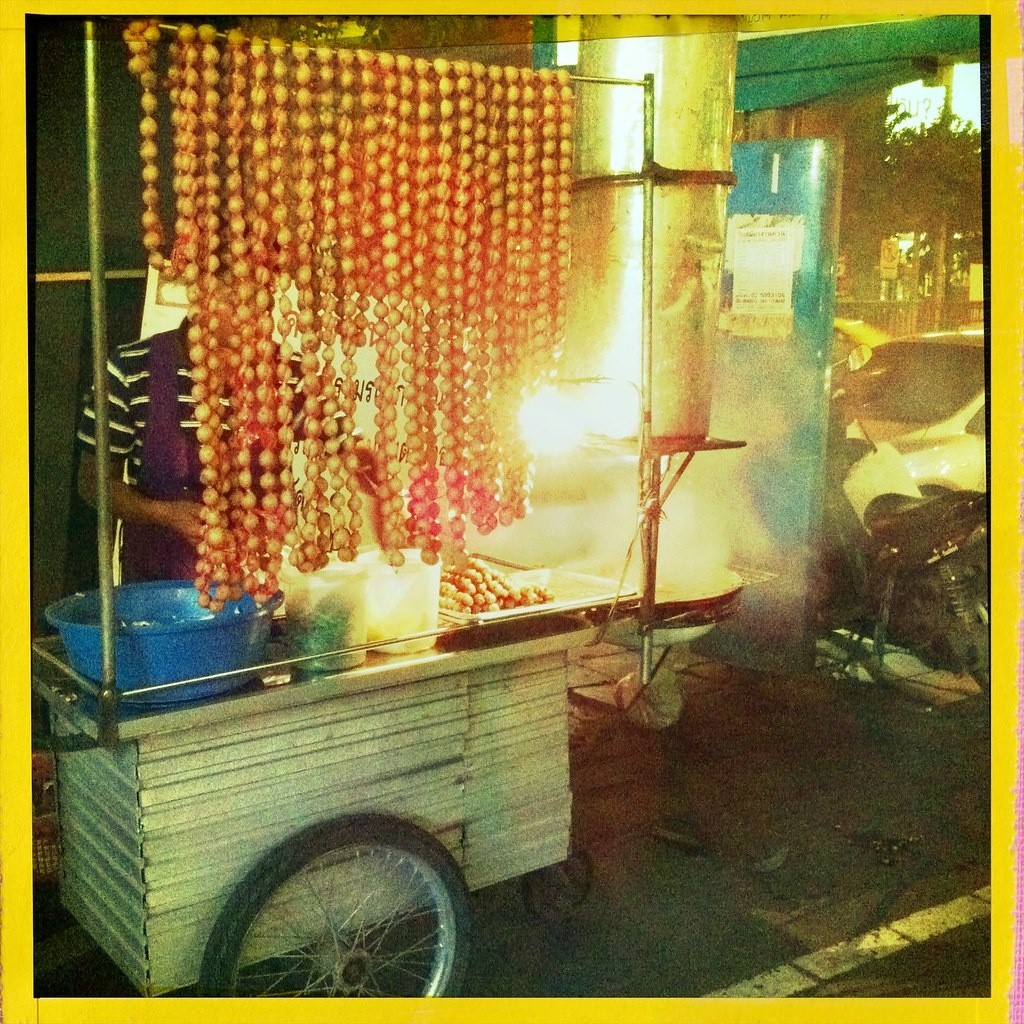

[352,543,444,657]
[277,545,368,673]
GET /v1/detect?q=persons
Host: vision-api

[825,328,858,450]
[75,246,382,583]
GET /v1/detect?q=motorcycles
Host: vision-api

[817,340,989,702]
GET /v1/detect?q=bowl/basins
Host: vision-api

[41,572,287,704]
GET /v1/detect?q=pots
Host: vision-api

[573,558,747,612]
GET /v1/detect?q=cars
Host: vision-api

[829,324,988,537]
